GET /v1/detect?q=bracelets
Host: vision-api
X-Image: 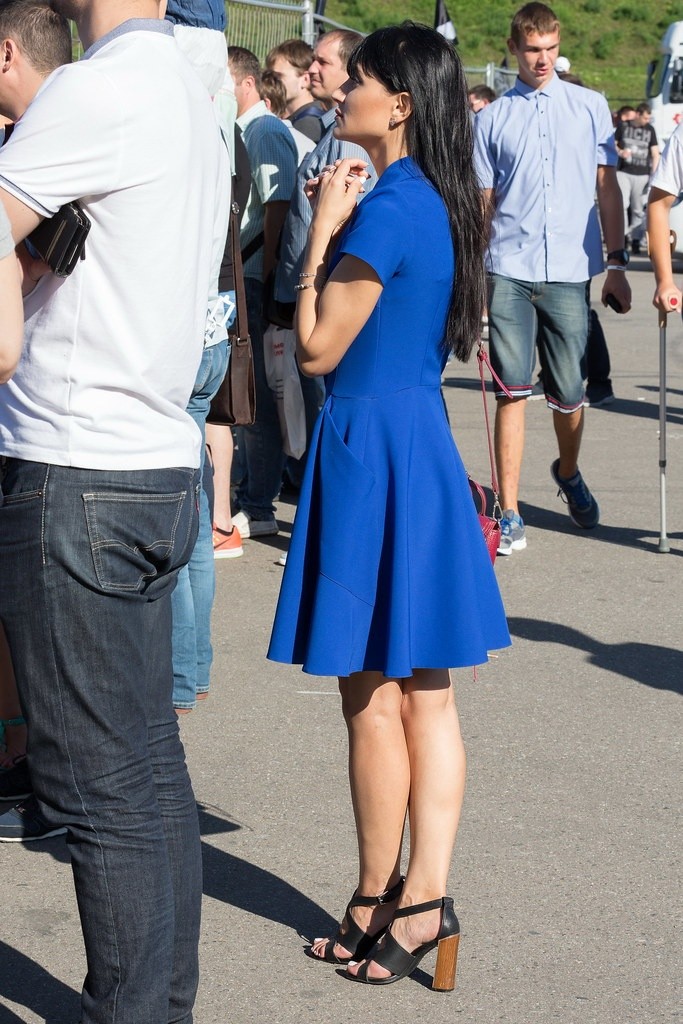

[294,284,324,290]
[608,266,626,272]
[299,273,326,278]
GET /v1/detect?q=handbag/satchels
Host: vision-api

[263,324,308,460]
[469,478,500,564]
[205,333,258,427]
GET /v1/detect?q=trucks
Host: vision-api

[644,20,683,252]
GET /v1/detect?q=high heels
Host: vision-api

[310,876,405,963]
[344,897,459,991]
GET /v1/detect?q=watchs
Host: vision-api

[607,249,629,264]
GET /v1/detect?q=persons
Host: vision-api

[470,84,497,330]
[0,0,229,1024]
[612,102,659,252]
[527,74,615,407]
[647,120,683,315]
[265,19,512,990]
[469,2,632,553]
[168,298,230,714]
[205,31,380,566]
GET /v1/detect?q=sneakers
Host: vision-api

[212,525,242,558]
[0,795,66,842]
[583,380,614,407]
[232,511,279,538]
[0,755,32,799]
[551,457,598,527]
[495,510,527,554]
[527,382,547,402]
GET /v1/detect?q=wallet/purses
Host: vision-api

[27,200,91,279]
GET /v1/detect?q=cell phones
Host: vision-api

[606,294,622,313]
